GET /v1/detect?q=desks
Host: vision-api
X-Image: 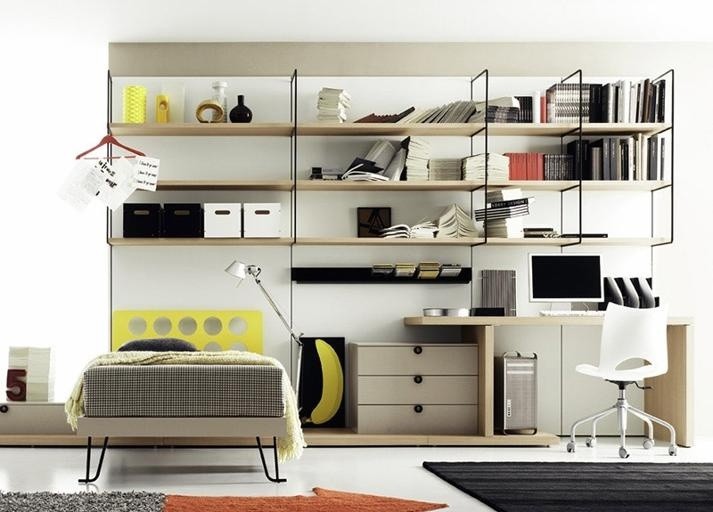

[404,315,691,447]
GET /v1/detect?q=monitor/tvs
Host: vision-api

[527,252,607,311]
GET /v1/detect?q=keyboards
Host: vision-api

[538,309,605,318]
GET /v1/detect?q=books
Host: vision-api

[567,132,665,180]
[354,96,533,124]
[534,78,666,123]
[316,87,353,124]
[379,189,556,239]
[302,77,667,241]
[310,136,573,181]
[370,259,464,279]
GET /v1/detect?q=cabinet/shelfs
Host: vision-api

[108,42,674,248]
[291,264,473,285]
[348,342,477,435]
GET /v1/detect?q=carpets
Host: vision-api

[422,460,713,512]
[0,486,450,512]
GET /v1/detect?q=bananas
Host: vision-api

[303,338,344,424]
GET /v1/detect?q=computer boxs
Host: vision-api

[493,350,539,436]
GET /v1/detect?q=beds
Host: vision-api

[77,309,289,485]
